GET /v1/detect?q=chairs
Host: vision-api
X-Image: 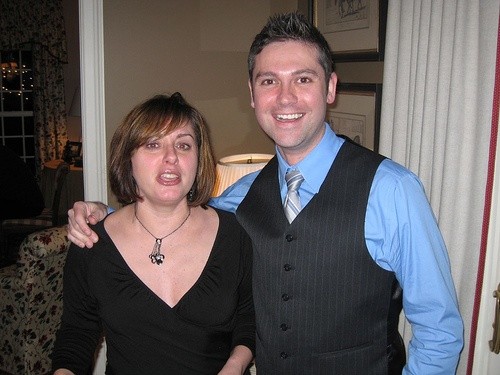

[0,188,74,375]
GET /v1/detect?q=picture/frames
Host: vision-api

[308,0,388,63]
[325,82,382,154]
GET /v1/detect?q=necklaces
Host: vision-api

[133,197,191,266]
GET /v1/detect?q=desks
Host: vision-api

[42,160,83,210]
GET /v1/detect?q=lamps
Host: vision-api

[208,153,276,197]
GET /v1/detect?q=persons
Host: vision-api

[42,93,257,375]
[66,17,465,375]
